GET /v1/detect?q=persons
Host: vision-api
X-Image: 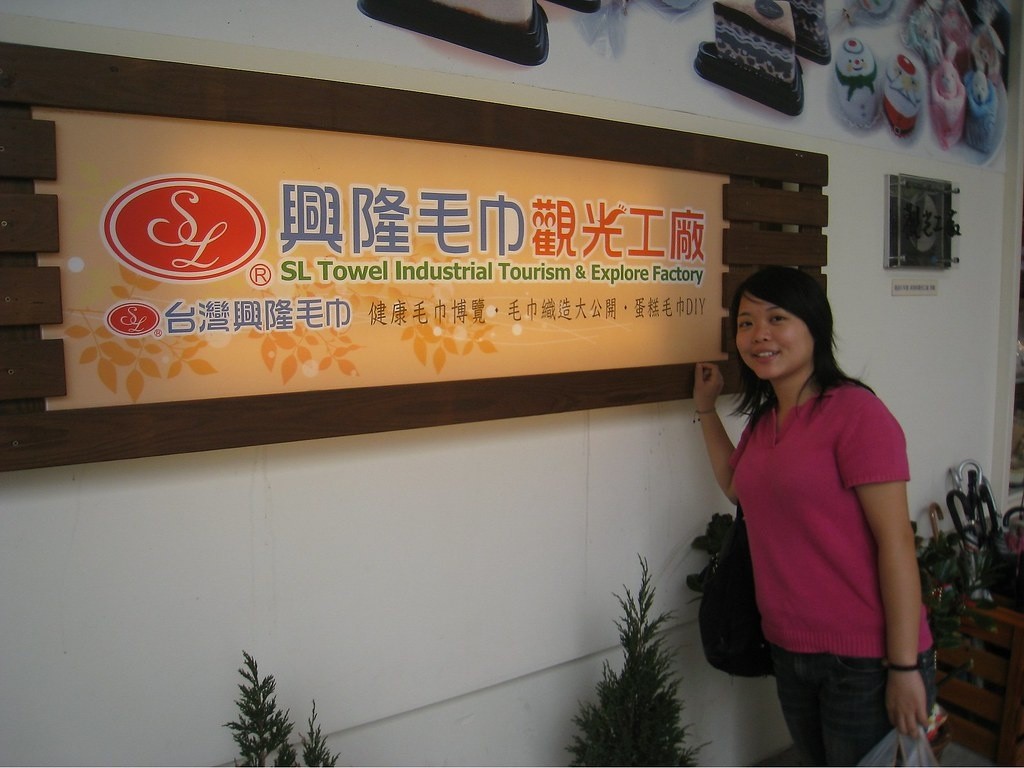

[693,266,936,767]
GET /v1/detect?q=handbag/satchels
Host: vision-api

[856,725,940,767]
[698,504,775,678]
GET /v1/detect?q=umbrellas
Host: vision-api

[912,459,1024,691]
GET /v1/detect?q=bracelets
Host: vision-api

[888,661,921,671]
[692,407,716,423]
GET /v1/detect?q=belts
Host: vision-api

[916,648,936,669]
